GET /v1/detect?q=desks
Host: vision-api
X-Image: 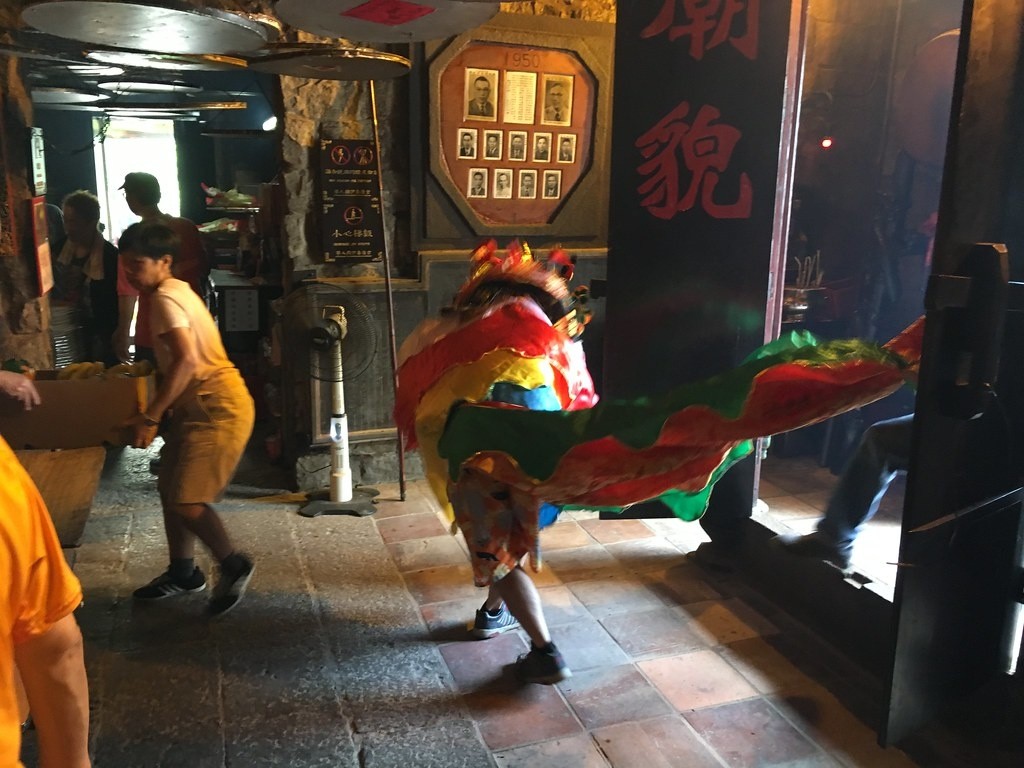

[14,445,107,548]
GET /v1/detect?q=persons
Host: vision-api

[47,189,129,461]
[536,137,550,160]
[511,135,525,159]
[114,219,258,625]
[468,76,496,116]
[235,158,289,276]
[472,172,486,196]
[546,174,559,197]
[560,137,574,161]
[0,367,41,412]
[521,174,536,197]
[546,83,569,122]
[447,471,570,686]
[777,370,921,572]
[461,132,474,156]
[497,173,511,196]
[487,134,500,157]
[116,171,215,474]
[1,434,91,768]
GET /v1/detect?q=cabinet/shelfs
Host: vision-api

[205,267,265,338]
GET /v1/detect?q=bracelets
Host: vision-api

[142,412,161,427]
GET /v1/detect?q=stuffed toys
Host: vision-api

[397,237,910,535]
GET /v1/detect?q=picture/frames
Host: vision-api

[541,169,562,199]
[455,127,478,160]
[466,167,489,199]
[507,130,528,162]
[462,66,499,123]
[532,131,553,163]
[482,129,504,160]
[557,133,578,164]
[540,72,575,127]
[518,168,539,200]
[492,167,514,200]
[501,69,538,125]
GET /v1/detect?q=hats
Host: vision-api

[117,172,160,191]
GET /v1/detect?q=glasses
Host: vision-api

[122,192,140,198]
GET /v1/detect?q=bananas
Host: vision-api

[56,360,153,379]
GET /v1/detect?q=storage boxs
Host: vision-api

[0,364,157,449]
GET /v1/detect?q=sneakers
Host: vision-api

[207,553,255,621]
[473,601,522,638]
[134,564,207,601]
[515,649,572,684]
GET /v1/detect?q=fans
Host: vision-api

[274,281,381,518]
